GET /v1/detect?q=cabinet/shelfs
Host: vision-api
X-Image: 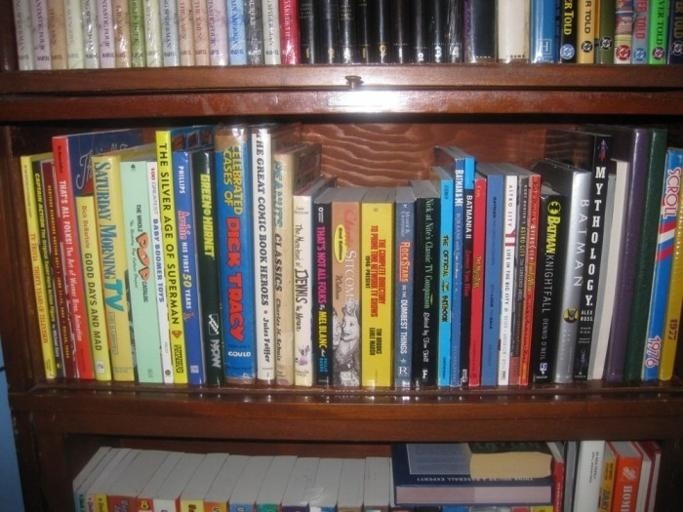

[0,0,683,512]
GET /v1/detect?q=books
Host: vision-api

[0,1,683,73]
[10,117,683,388]
[68,438,666,512]
